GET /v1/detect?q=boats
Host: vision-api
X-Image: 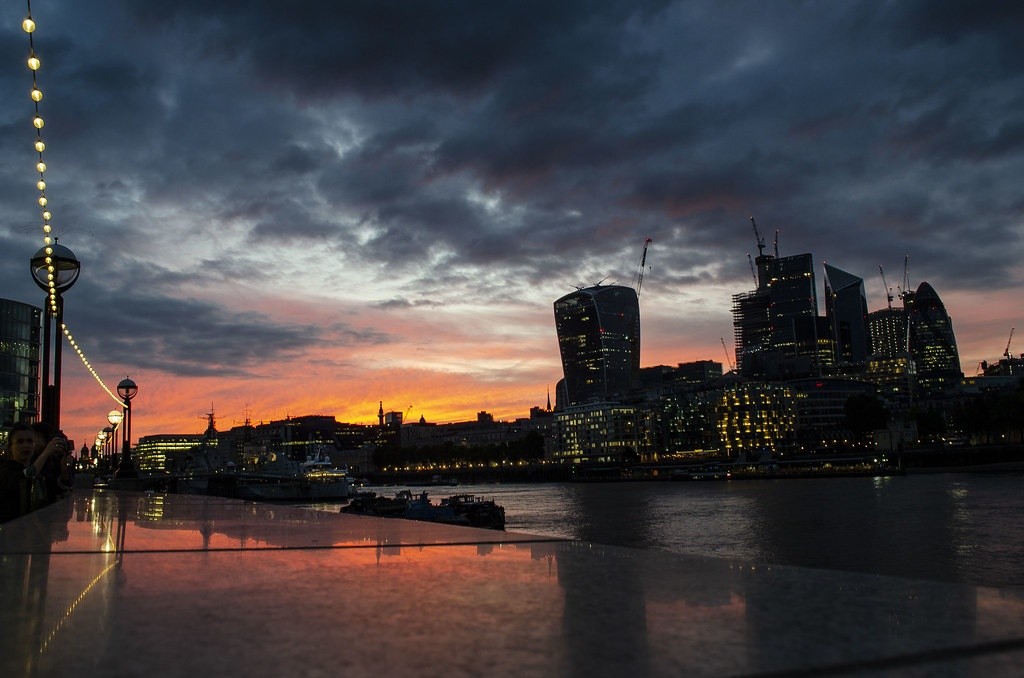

[340,488,507,532]
[155,404,354,504]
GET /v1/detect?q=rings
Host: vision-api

[56,444,59,447]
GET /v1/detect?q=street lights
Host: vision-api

[92,487,116,554]
[95,425,113,472]
[30,235,81,511]
[107,408,122,476]
[117,376,138,478]
[107,485,130,589]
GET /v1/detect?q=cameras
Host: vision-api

[54,437,74,461]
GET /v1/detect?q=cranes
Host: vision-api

[898,253,912,300]
[751,216,766,256]
[879,265,895,309]
[1003,326,1014,359]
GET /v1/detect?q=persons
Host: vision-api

[0,421,75,609]
